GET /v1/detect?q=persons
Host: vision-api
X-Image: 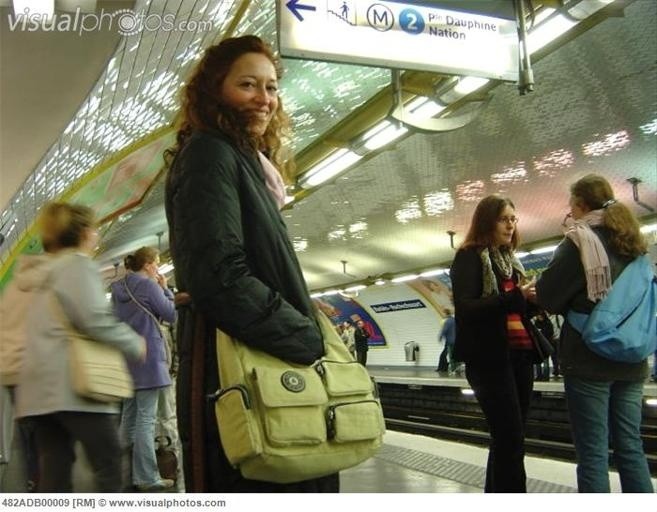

[162,34,342,493]
[534,172,657,493]
[528,311,565,382]
[433,309,456,373]
[451,194,539,493]
[334,319,370,367]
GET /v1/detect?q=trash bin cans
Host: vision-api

[404,341,419,362]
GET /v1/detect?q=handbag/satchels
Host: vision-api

[583,255,657,363]
[69,339,135,403]
[215,308,386,481]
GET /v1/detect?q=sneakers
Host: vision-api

[137,478,175,490]
